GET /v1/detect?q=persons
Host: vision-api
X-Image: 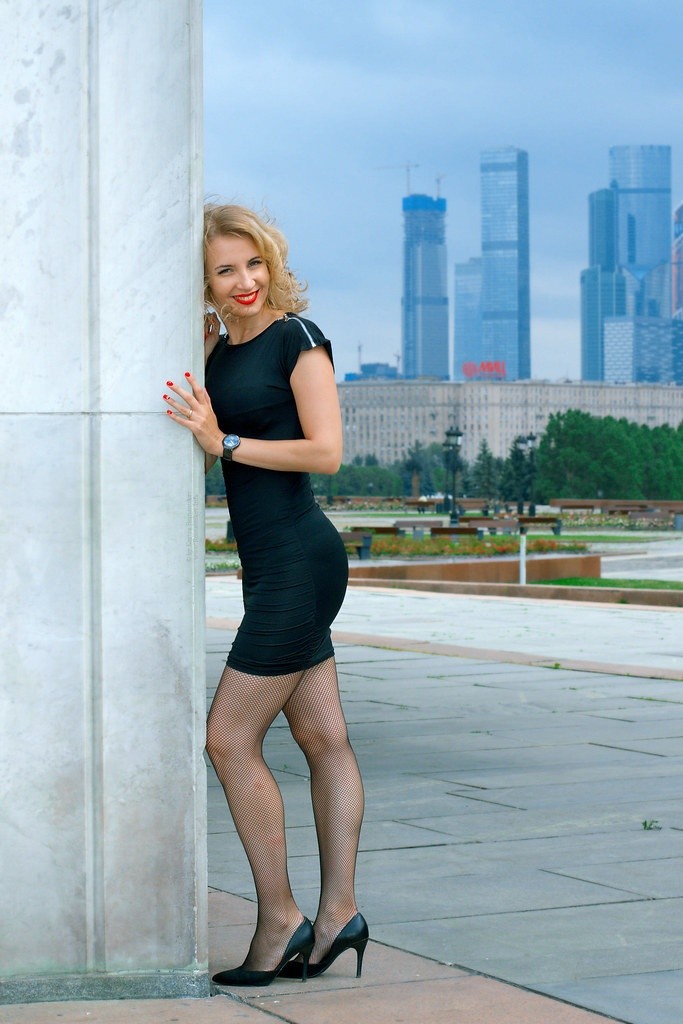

[162,202,370,987]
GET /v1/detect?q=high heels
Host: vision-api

[212,917,316,987]
[278,913,369,979]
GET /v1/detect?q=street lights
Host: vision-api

[450,426,463,526]
[442,425,455,513]
[516,435,526,515]
[527,431,537,516]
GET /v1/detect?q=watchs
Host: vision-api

[220,434,241,461]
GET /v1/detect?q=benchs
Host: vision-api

[608,507,671,530]
[225,516,563,560]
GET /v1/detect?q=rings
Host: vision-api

[187,409,193,418]
